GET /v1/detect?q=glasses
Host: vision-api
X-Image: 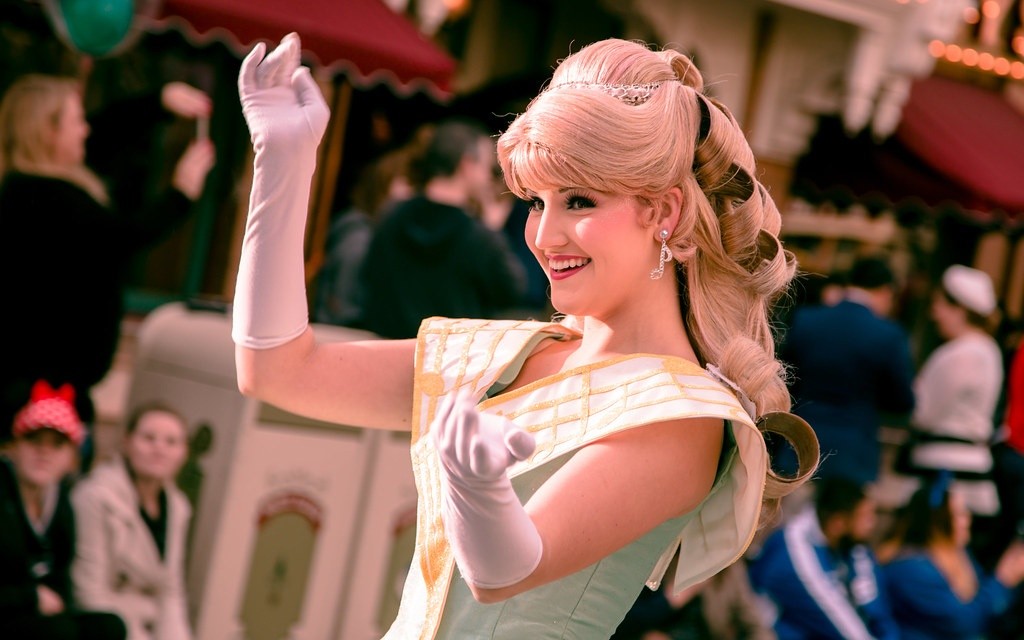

[19,428,68,448]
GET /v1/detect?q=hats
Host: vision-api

[14,380,86,447]
[942,265,997,317]
[849,255,891,290]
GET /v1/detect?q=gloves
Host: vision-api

[231,33,330,351]
[429,393,543,590]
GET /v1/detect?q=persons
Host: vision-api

[0,74,216,469]
[911,266,1003,548]
[230,33,818,640]
[749,479,905,640]
[67,406,195,640]
[608,527,782,640]
[776,254,915,521]
[314,121,552,342]
[0,381,127,640]
[881,484,1024,640]
